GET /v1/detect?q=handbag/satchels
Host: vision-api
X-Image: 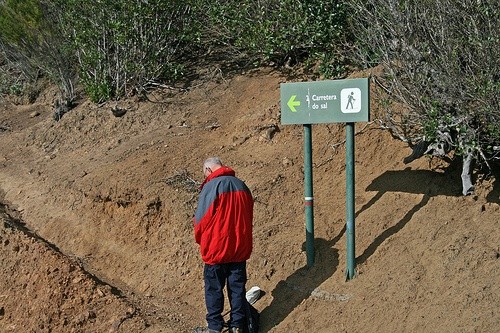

[245,298,260,333]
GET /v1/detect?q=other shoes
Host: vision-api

[196,326,221,333]
[229,328,243,333]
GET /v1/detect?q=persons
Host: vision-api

[194,157,254,333]
